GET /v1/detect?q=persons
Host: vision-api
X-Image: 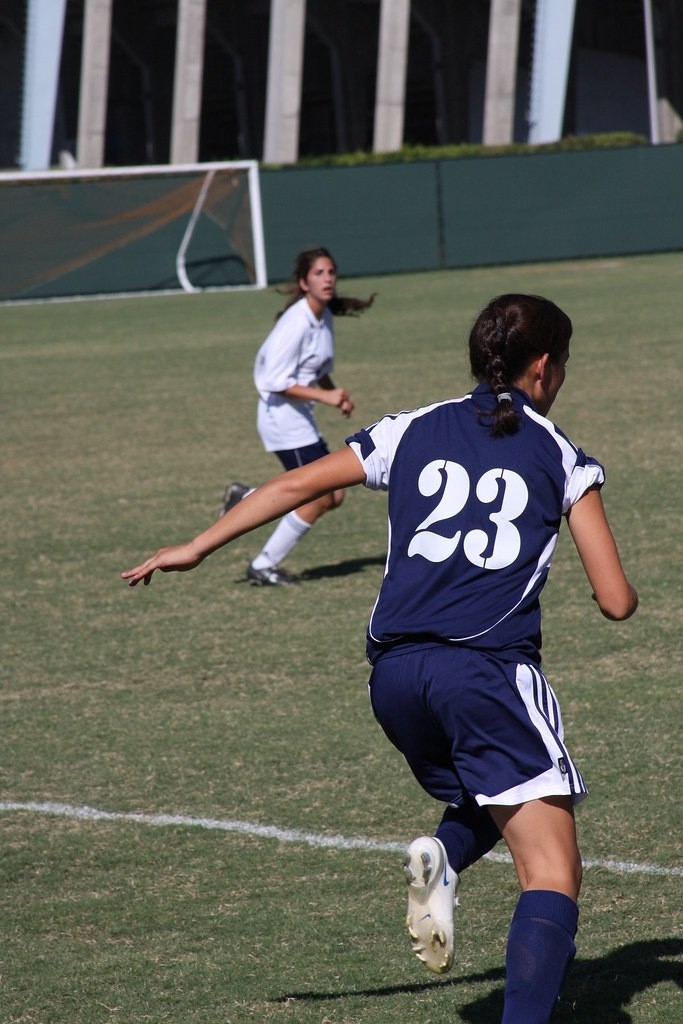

[218,247,377,586]
[121,293,638,1024]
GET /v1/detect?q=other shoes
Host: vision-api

[404,835,460,974]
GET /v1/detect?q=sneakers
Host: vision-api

[246,560,295,586]
[219,483,249,516]
